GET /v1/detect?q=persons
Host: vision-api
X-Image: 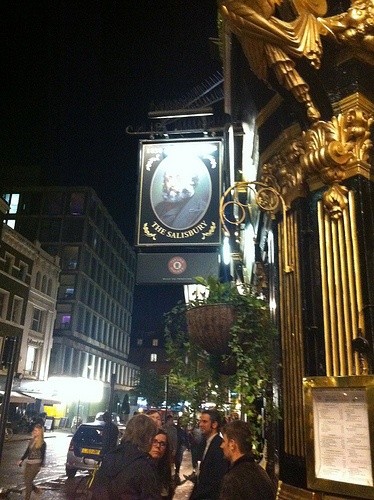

[218,421,277,500]
[146,410,202,473]
[86,414,164,500]
[17,424,46,500]
[147,429,173,500]
[101,411,118,465]
[188,410,230,500]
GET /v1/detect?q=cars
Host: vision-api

[66,421,127,478]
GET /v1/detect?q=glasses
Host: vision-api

[153,439,168,449]
